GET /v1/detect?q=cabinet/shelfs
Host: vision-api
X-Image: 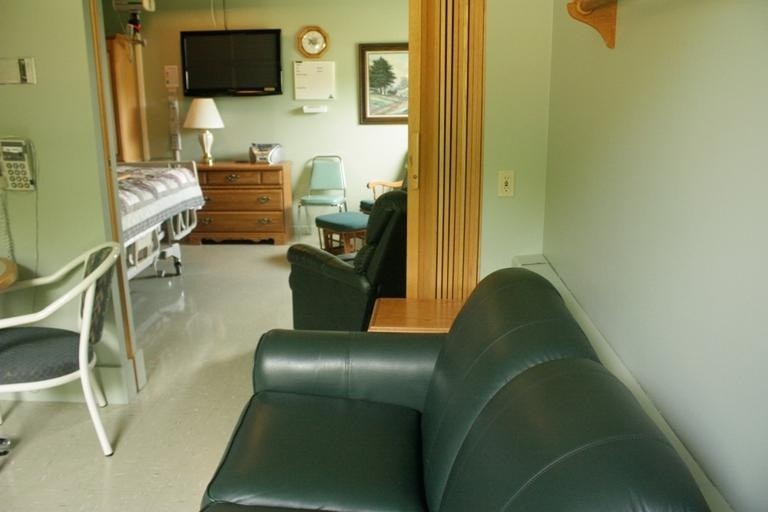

[187,160,294,246]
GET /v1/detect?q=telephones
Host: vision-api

[0,137,35,192]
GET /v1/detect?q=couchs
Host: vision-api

[286,189,407,332]
[195,266,712,512]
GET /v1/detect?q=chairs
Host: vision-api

[0,240,124,458]
[367,152,409,201]
[298,154,347,237]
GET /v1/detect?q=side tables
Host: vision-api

[367,296,465,334]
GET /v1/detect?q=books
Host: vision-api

[118,174,131,180]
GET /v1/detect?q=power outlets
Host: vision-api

[497,170,514,197]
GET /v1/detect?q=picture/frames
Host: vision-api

[358,43,409,125]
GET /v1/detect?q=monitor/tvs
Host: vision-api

[180,28,282,97]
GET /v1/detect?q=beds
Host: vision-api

[116,159,206,282]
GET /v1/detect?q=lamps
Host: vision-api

[182,97,224,168]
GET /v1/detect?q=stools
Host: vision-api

[314,211,370,255]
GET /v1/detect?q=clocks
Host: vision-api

[296,24,330,59]
